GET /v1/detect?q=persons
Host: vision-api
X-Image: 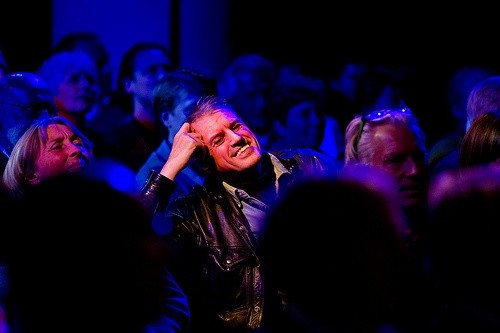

[0,31,500,333]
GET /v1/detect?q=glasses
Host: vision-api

[354,107,411,156]
